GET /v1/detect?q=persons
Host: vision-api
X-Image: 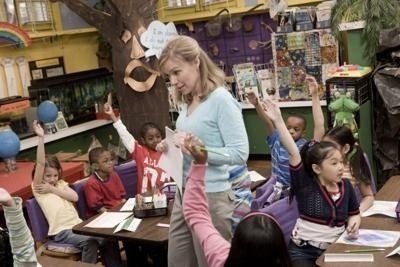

[179,129,296,266]
[30,119,105,262]
[156,33,251,265]
[306,75,375,212]
[81,145,126,218]
[260,97,361,266]
[0,187,38,266]
[104,101,174,195]
[245,90,314,198]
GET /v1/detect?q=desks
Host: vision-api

[72,167,273,267]
[316,175,400,267]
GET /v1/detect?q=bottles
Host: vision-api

[94,100,104,113]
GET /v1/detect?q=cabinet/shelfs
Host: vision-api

[0,0,62,39]
[157,0,242,23]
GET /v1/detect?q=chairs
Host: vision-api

[114,159,137,200]
[25,196,83,261]
[252,174,362,248]
[71,177,90,220]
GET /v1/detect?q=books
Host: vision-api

[269,28,342,102]
[156,124,185,198]
[223,61,277,106]
[277,0,337,34]
[42,110,69,132]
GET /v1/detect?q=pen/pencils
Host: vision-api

[193,146,229,158]
[344,248,386,253]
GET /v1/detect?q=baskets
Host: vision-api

[160,185,176,199]
[132,200,168,217]
[326,77,370,105]
